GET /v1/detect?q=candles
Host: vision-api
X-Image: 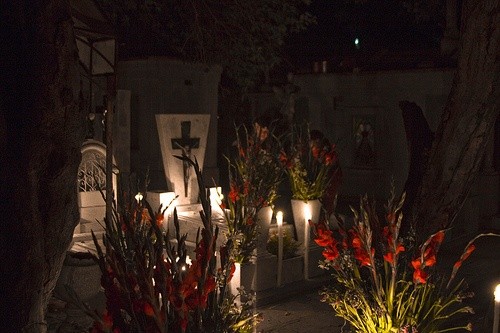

[302,203,312,280]
[276,211,283,287]
[493,284,500,333]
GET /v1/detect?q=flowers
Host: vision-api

[79,119,500,333]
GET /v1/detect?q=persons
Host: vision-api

[254,116,342,212]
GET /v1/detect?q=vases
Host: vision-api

[291,199,321,242]
